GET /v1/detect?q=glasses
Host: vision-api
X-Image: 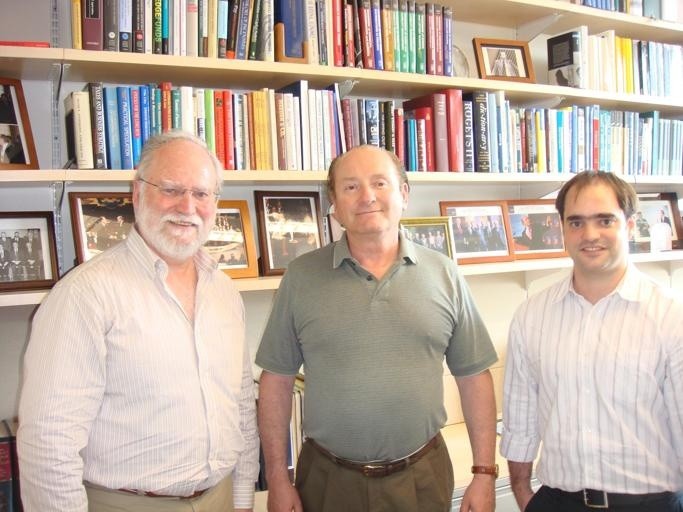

[135,175,216,202]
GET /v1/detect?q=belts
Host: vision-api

[122,487,208,502]
[304,430,443,478]
[569,488,674,509]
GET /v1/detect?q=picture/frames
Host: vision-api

[253,190,325,276]
[68,191,143,285]
[189,199,258,279]
[399,219,457,268]
[0,210,62,291]
[471,37,538,85]
[508,199,570,259]
[622,196,681,252]
[441,200,516,265]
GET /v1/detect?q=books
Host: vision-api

[63,1,453,78]
[64,79,683,177]
[547,25,683,96]
[575,0,683,23]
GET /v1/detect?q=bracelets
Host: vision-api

[470,464,500,479]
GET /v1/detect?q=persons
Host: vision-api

[498,169,682,512]
[413,215,500,252]
[490,50,519,76]
[106,215,130,240]
[250,142,502,512]
[94,215,108,250]
[0,229,40,281]
[16,130,259,512]
[659,209,671,227]
[636,212,650,237]
[511,216,551,251]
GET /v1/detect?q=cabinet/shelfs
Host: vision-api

[1,0,681,310]
